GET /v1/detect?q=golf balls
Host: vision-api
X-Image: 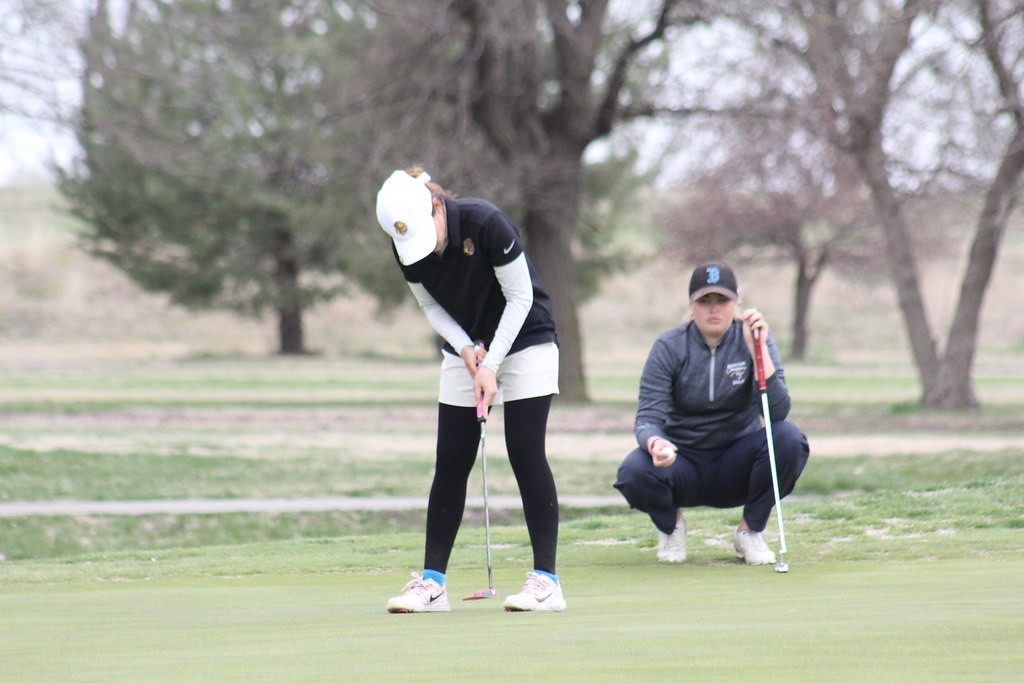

[661,444,676,462]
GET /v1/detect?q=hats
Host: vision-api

[374,169,438,267]
[689,262,738,306]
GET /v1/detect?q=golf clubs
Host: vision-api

[460,344,498,601]
[748,317,790,574]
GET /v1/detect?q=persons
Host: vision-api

[375,164,568,614]
[613,261,810,565]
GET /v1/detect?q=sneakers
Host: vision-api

[656,514,687,562]
[386,572,451,612]
[733,526,776,565]
[503,572,566,611]
